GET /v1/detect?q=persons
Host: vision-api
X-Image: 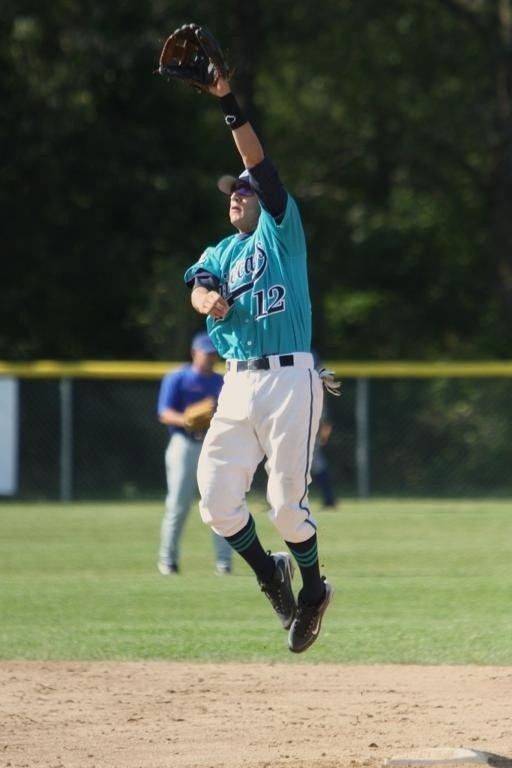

[157,19,333,653]
[302,342,338,505]
[155,331,232,578]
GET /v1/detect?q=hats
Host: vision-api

[218,167,265,193]
[191,332,216,354]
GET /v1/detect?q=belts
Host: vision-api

[228,355,294,372]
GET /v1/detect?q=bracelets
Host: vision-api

[216,90,248,131]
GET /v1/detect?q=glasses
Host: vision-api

[230,184,256,199]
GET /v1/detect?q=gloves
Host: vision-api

[318,367,343,398]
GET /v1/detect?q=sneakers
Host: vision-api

[156,559,178,574]
[216,566,230,574]
[257,550,297,630]
[287,575,335,653]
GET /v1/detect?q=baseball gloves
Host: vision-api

[159,23,229,87]
[183,396,216,437]
